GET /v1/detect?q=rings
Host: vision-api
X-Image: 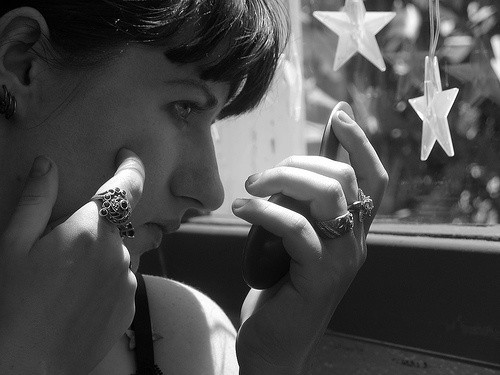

[346,188,374,222]
[89,187,135,240]
[308,210,353,239]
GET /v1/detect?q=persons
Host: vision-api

[0,1,390,375]
